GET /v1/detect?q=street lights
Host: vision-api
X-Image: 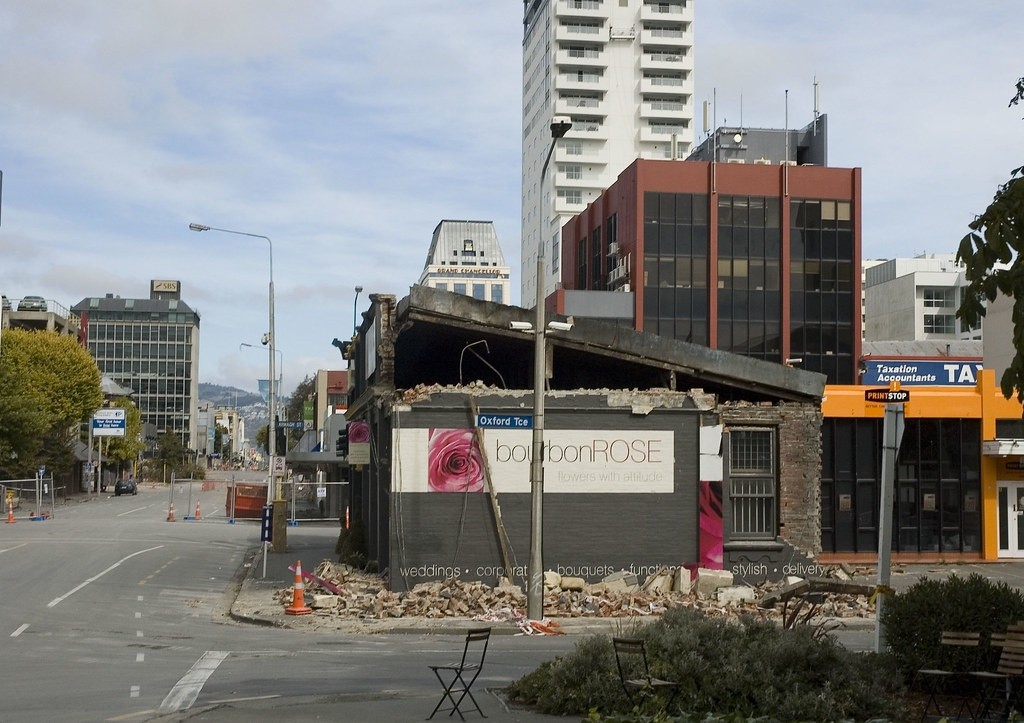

[524,114,574,623]
[239,342,284,456]
[352,284,364,335]
[188,222,277,553]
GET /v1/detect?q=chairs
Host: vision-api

[917,629,983,723]
[988,632,1024,723]
[425,626,492,722]
[612,637,686,718]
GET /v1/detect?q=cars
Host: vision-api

[114,478,138,496]
[2,296,13,310]
[17,296,49,312]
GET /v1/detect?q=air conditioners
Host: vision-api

[614,289,618,292]
[780,160,796,166]
[622,283,630,292]
[612,268,616,280]
[608,271,612,281]
[727,159,745,164]
[753,160,772,164]
[616,266,626,278]
[609,241,619,253]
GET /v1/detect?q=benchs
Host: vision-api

[956,624,1024,723]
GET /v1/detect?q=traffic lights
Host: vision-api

[335,439,348,460]
[338,422,350,455]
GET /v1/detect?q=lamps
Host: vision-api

[1013,440,1019,446]
[1002,455,1007,458]
[618,287,622,292]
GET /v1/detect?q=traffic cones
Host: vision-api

[4,500,17,523]
[195,498,203,520]
[285,559,313,615]
[165,501,175,522]
